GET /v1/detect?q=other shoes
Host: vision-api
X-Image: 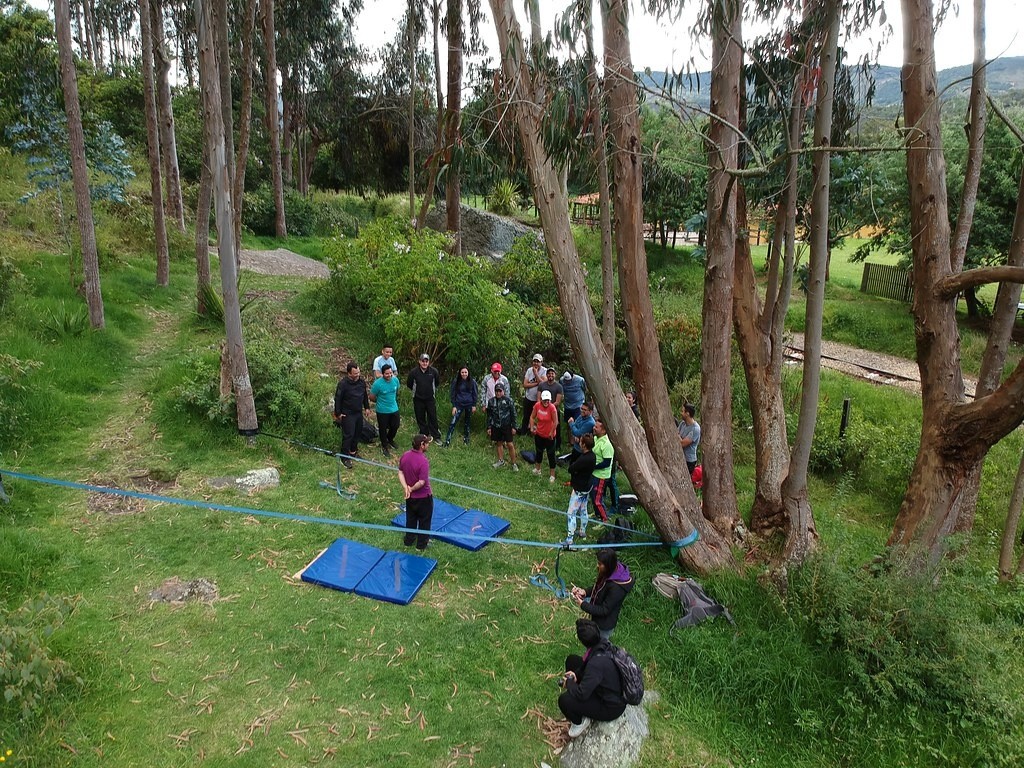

[444,439,450,447]
[463,438,468,445]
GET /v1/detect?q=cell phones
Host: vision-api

[571,593,578,600]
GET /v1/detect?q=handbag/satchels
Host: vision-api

[596,517,635,551]
[617,493,639,517]
[556,451,573,467]
[520,450,536,464]
[691,465,704,488]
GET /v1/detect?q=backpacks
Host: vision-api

[650,573,738,638]
[596,637,644,706]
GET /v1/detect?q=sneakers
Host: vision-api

[434,439,443,445]
[492,459,505,468]
[592,524,606,531]
[511,464,519,472]
[388,441,399,449]
[340,458,352,469]
[563,481,571,486]
[349,451,363,462]
[381,446,392,458]
[557,541,576,546]
[577,533,587,540]
[549,475,556,483]
[568,717,591,738]
[588,512,596,519]
[532,468,541,475]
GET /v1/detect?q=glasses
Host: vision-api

[580,407,590,411]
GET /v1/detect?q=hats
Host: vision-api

[563,370,573,381]
[494,383,505,390]
[540,390,552,401]
[533,353,543,361]
[491,363,502,372]
[420,353,430,361]
[547,367,556,372]
[412,434,433,447]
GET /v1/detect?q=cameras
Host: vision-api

[558,674,574,687]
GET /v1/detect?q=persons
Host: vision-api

[368,364,401,457]
[569,546,636,642]
[482,352,637,548]
[372,345,398,381]
[397,433,434,550]
[676,403,702,476]
[443,367,479,446]
[557,618,627,739]
[406,354,445,446]
[333,361,373,469]
